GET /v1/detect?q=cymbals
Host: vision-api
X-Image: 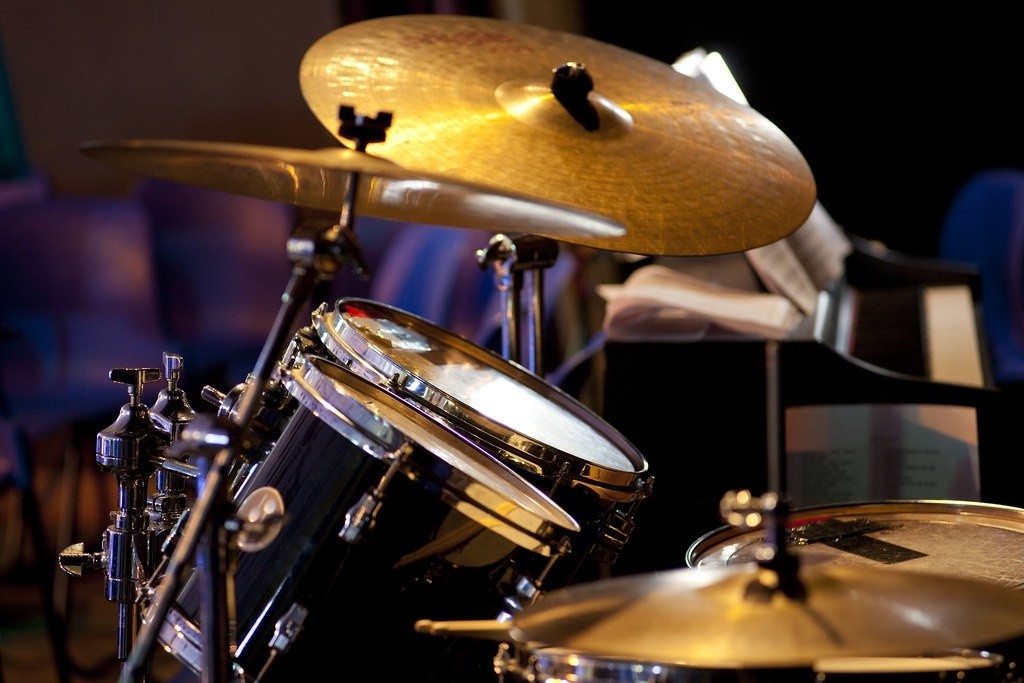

[76,139,628,238]
[512,568,1024,664]
[299,15,819,258]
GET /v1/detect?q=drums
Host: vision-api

[137,351,582,683]
[492,642,1024,683]
[683,497,1024,591]
[300,296,656,597]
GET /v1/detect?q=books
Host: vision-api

[596,47,854,342]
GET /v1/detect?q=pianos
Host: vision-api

[599,238,1001,567]
